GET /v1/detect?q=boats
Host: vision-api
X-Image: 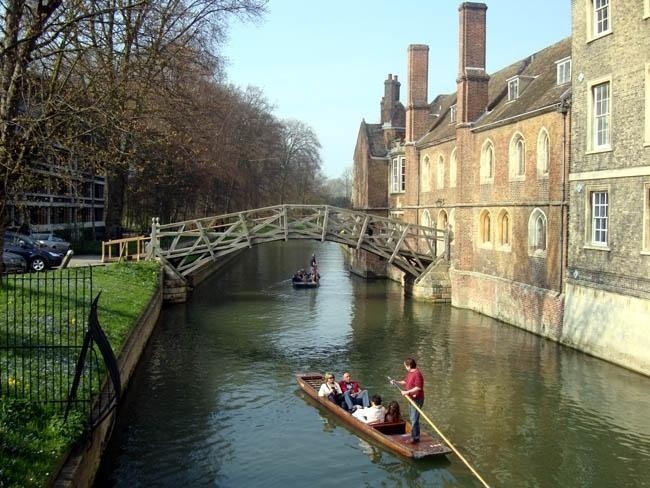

[292,273,319,284]
[296,373,453,459]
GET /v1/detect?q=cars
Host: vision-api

[0,230,72,276]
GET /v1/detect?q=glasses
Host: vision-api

[327,377,335,379]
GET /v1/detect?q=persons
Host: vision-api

[318,372,354,411]
[365,216,376,244]
[293,252,320,284]
[18,221,34,239]
[337,372,371,414]
[350,394,386,425]
[388,357,425,445]
[384,401,403,422]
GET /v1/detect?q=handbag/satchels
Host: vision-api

[328,391,338,402]
